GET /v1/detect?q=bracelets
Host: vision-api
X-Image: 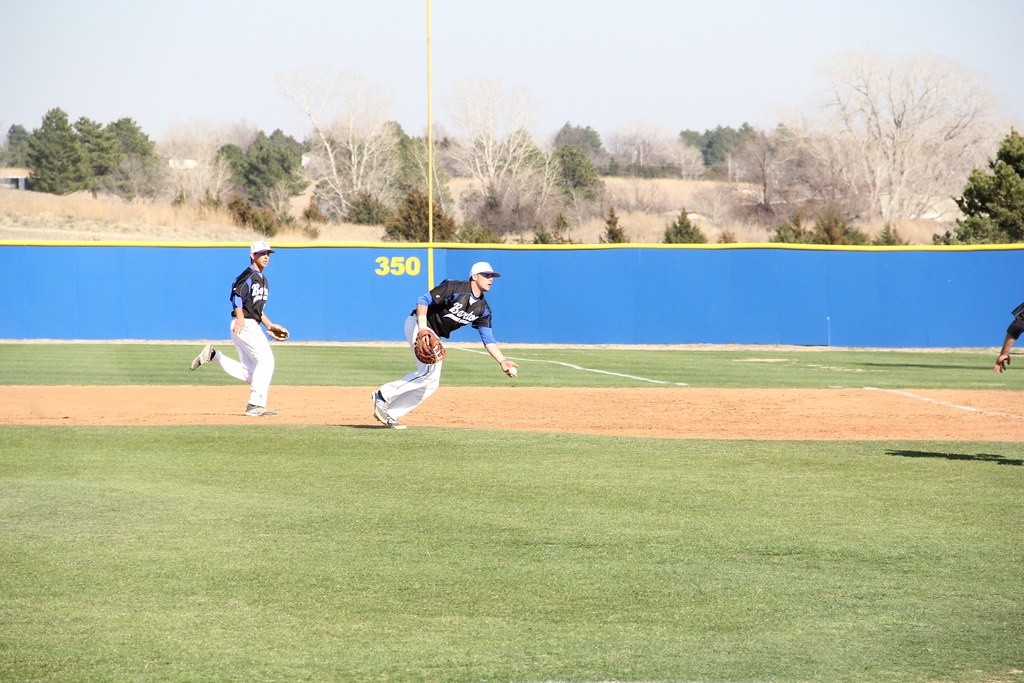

[500,360,507,365]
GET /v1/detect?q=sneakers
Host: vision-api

[245,404,277,416]
[371,390,388,424]
[190,344,213,370]
[387,418,406,429]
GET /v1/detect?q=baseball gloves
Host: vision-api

[266,324,290,341]
[414,329,446,364]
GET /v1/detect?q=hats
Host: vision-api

[469,262,500,280]
[250,241,275,255]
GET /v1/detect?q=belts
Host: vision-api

[410,312,414,316]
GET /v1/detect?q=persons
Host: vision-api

[371,261,519,428]
[191,240,289,417]
[994,302,1024,375]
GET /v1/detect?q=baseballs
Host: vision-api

[509,367,517,376]
